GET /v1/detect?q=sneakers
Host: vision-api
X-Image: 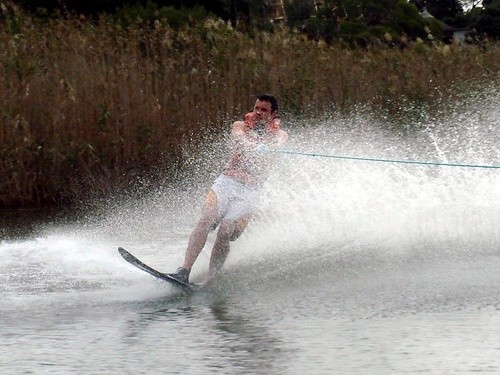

[175,268,192,280]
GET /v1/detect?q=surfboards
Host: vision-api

[118,247,196,294]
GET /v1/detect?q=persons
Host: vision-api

[162,94,289,284]
